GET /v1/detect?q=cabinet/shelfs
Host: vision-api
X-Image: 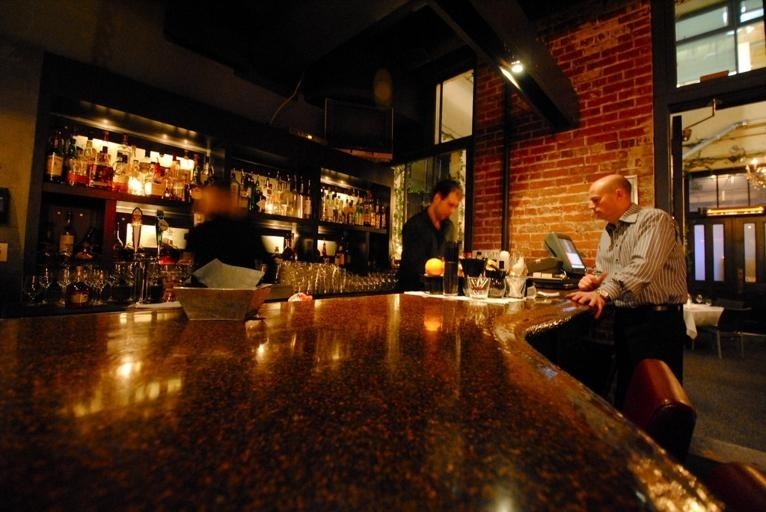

[21,51,393,268]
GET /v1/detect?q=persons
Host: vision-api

[186,169,278,290]
[388,175,467,292]
[562,171,689,411]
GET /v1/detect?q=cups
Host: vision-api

[696,294,713,307]
[274,260,402,293]
[468,276,491,299]
[488,277,507,297]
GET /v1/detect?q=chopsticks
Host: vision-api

[443,240,459,263]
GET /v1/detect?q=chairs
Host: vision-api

[622,350,696,466]
[703,307,752,358]
[697,299,746,344]
[702,461,766,512]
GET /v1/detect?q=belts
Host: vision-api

[645,303,682,311]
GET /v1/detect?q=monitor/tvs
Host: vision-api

[543,233,586,275]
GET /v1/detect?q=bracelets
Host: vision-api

[599,288,611,301]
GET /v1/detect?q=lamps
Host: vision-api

[494,42,523,73]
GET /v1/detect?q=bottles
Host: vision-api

[321,243,330,264]
[281,233,299,261]
[21,263,191,307]
[47,123,390,230]
[112,218,124,262]
[38,207,103,263]
[302,241,319,263]
[334,239,346,267]
